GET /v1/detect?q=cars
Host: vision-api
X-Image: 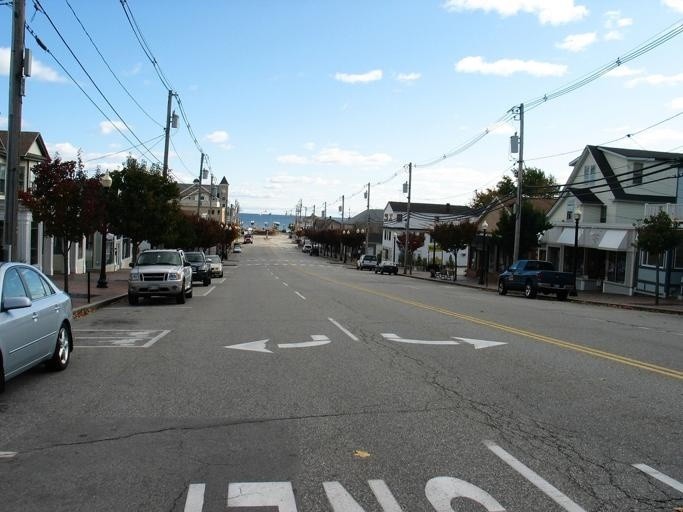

[0,260,74,389]
[373,260,399,274]
[204,254,223,279]
[232,244,241,253]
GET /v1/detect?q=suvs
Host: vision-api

[182,251,212,286]
[355,254,377,272]
[301,245,311,253]
[243,235,253,244]
[125,247,194,307]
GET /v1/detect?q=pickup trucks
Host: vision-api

[496,259,576,301]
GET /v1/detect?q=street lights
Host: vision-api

[342,229,351,263]
[96,167,112,289]
[568,209,581,297]
[355,228,364,253]
[478,220,488,285]
